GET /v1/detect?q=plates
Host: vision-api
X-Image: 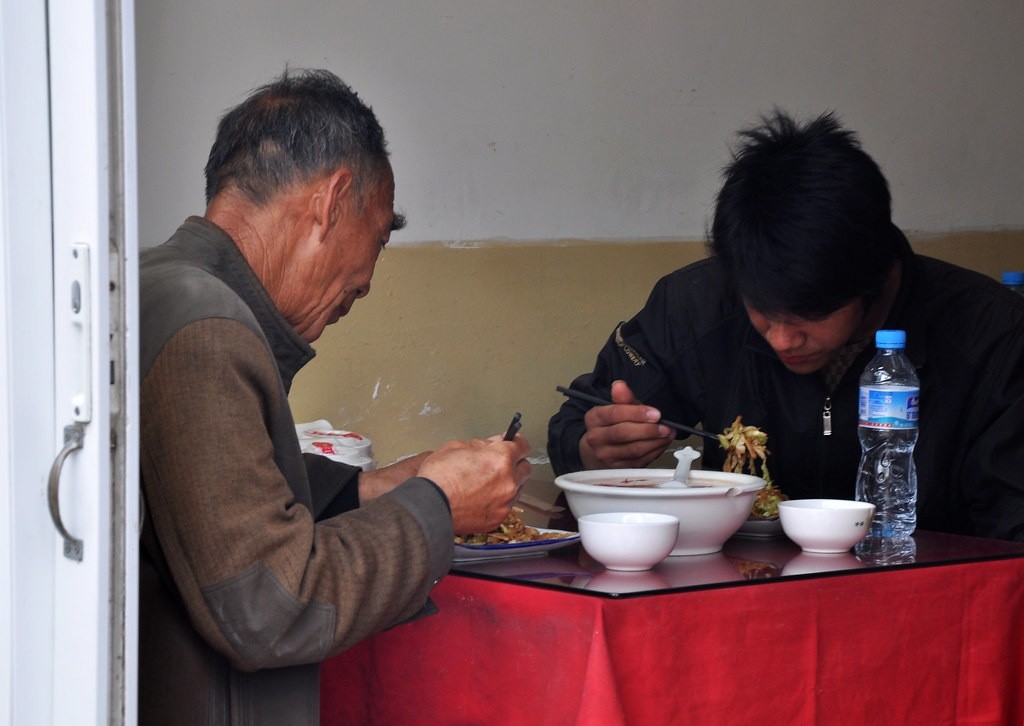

[452,526,581,563]
[733,520,784,537]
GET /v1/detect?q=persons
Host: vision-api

[546,110,1024,536]
[138,69,533,726]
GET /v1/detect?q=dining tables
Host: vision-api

[320,480,1024,726]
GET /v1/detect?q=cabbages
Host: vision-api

[721,424,788,518]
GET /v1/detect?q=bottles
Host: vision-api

[1000,271,1024,295]
[853,329,921,566]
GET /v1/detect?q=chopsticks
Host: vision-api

[503,412,522,441]
[555,384,721,441]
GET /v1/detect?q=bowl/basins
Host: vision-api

[778,498,876,554]
[554,467,768,556]
[578,512,680,571]
